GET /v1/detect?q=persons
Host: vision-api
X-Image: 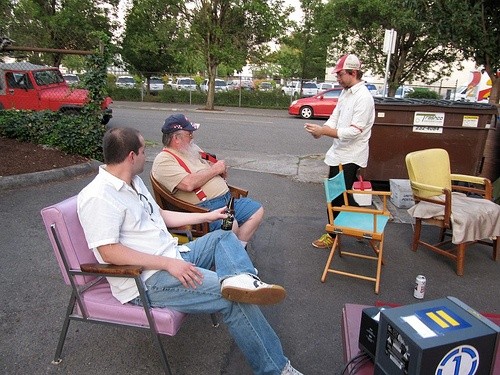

[76,126,304,375]
[152,114,264,275]
[305,54,375,248]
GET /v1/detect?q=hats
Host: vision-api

[161,114,200,134]
[331,54,361,74]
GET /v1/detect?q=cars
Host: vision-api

[199,78,229,95]
[281,81,318,98]
[229,80,252,91]
[287,86,345,119]
[115,75,136,89]
[142,77,164,90]
[169,77,198,92]
[318,82,417,99]
[63,74,80,87]
[259,81,274,91]
[445,84,480,102]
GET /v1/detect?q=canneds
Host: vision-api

[414,275,426,299]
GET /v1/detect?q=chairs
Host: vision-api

[321,164,393,295]
[149,171,248,237]
[404,148,500,276]
[41,195,220,375]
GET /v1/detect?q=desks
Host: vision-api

[341,303,500,375]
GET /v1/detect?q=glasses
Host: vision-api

[177,131,193,139]
[335,71,349,78]
[138,194,154,215]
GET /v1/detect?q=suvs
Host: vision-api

[0,60,114,125]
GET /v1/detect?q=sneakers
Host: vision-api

[312,233,339,248]
[220,273,286,304]
[281,361,305,375]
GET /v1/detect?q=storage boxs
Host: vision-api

[389,178,416,208]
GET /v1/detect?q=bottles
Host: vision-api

[221,196,235,230]
